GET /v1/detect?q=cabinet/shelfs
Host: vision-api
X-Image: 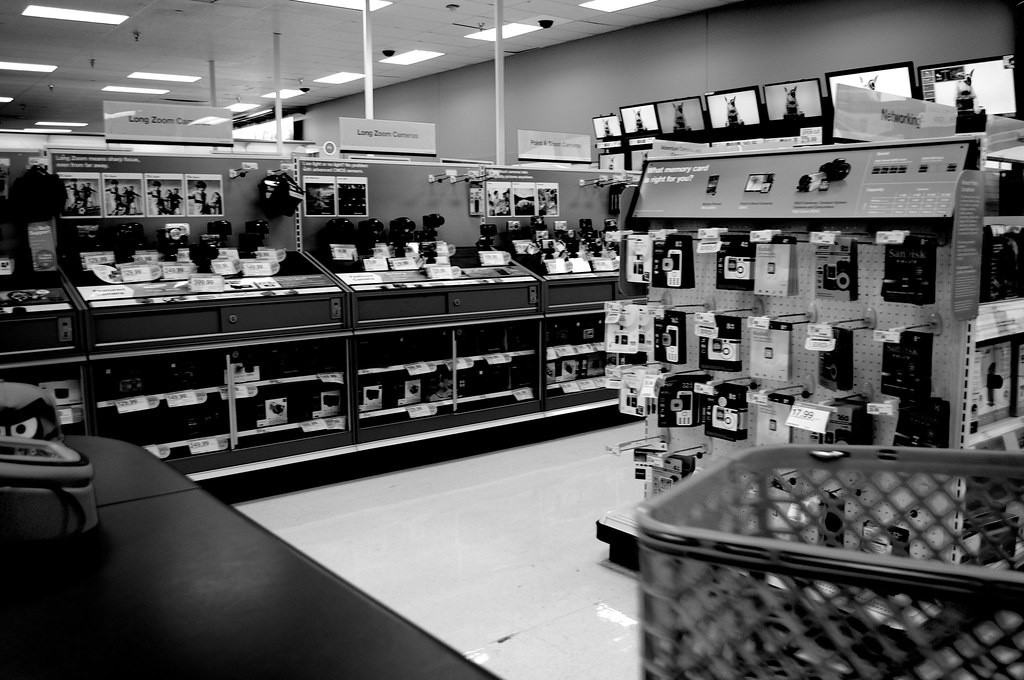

[0,147,648,506]
[595,143,1024,576]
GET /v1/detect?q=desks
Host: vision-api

[1,437,505,680]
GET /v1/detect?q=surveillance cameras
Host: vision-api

[299,88,310,92]
[538,20,554,28]
[382,50,395,57]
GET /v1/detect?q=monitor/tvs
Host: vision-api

[590,52,1020,183]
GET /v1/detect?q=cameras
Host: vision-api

[725,257,751,279]
[115,222,144,253]
[77,225,103,252]
[529,217,619,261]
[156,227,188,252]
[268,359,605,416]
[820,158,851,180]
[189,244,220,269]
[207,219,233,238]
[708,338,739,361]
[713,406,738,432]
[508,221,522,231]
[246,220,271,236]
[480,223,498,237]
[328,214,445,258]
[744,174,774,193]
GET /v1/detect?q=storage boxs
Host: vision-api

[969,222,1024,435]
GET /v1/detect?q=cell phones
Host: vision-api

[706,174,719,195]
[667,250,683,287]
[676,391,694,426]
[666,325,678,362]
[825,258,852,292]
